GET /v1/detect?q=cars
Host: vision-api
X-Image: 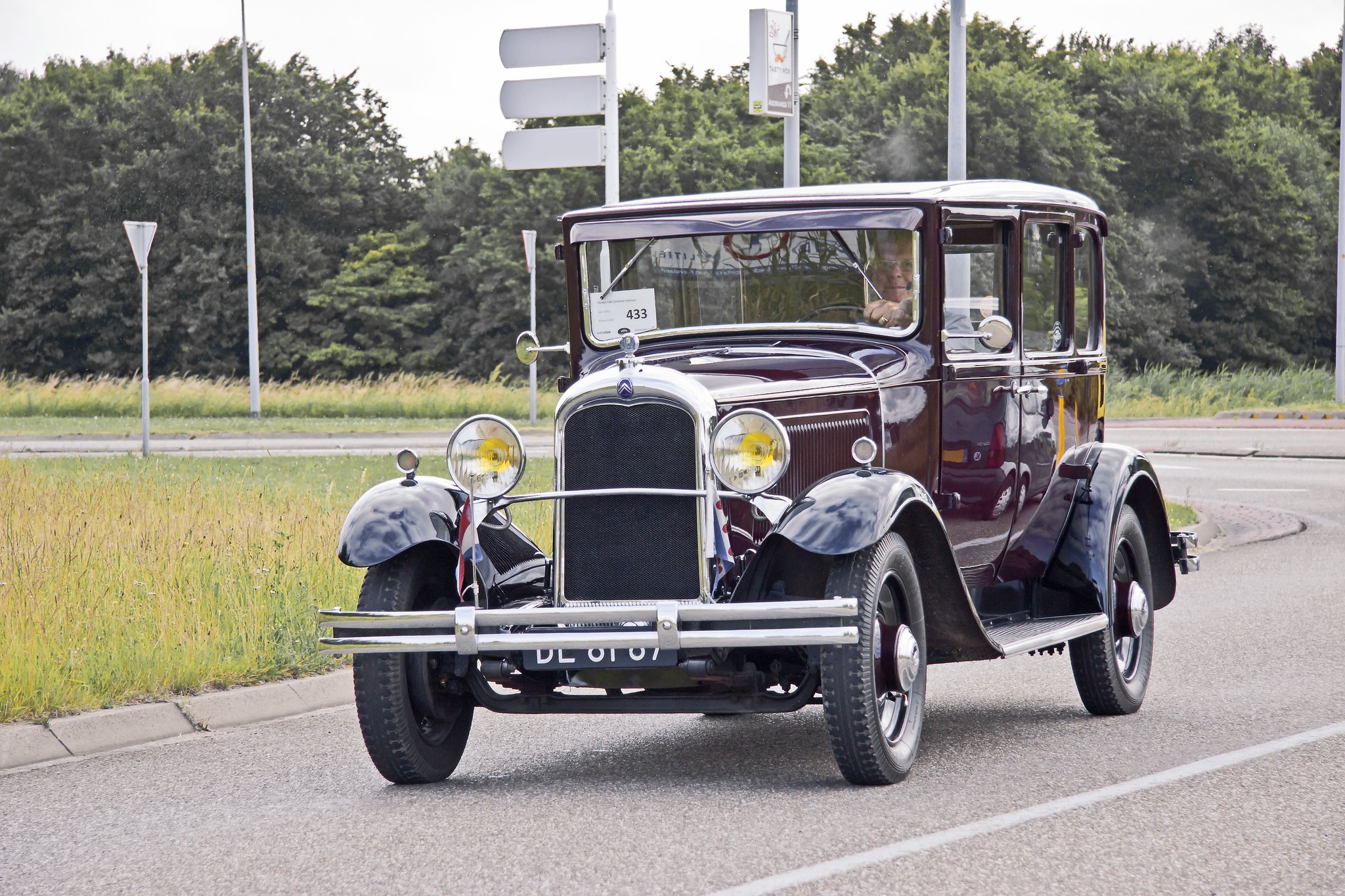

[315,179,1205,790]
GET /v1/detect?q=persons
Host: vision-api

[856,229,975,355]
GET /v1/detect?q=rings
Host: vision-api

[881,316,889,324]
